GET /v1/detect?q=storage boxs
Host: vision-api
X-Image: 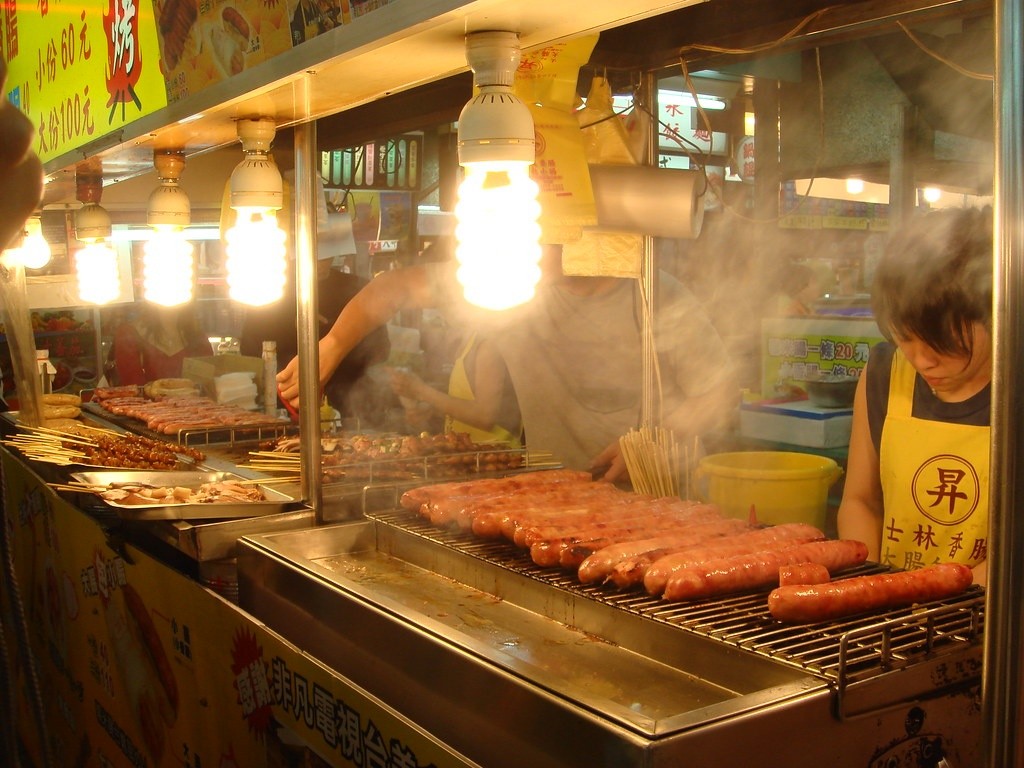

[182,354,264,403]
[760,313,889,383]
[743,436,854,508]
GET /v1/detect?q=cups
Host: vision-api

[357,202,371,227]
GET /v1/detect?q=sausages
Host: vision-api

[94,376,290,436]
[42,393,120,440]
[400,469,975,622]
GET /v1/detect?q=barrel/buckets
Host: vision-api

[698,451,844,534]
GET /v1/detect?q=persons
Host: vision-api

[277,247,744,486]
[240,257,391,420]
[836,204,991,592]
[382,321,522,447]
[781,263,821,317]
[112,301,215,391]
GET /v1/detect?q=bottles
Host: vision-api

[320,396,333,433]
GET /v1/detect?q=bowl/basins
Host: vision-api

[806,377,858,406]
[72,369,97,382]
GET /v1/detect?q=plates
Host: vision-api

[53,363,73,393]
